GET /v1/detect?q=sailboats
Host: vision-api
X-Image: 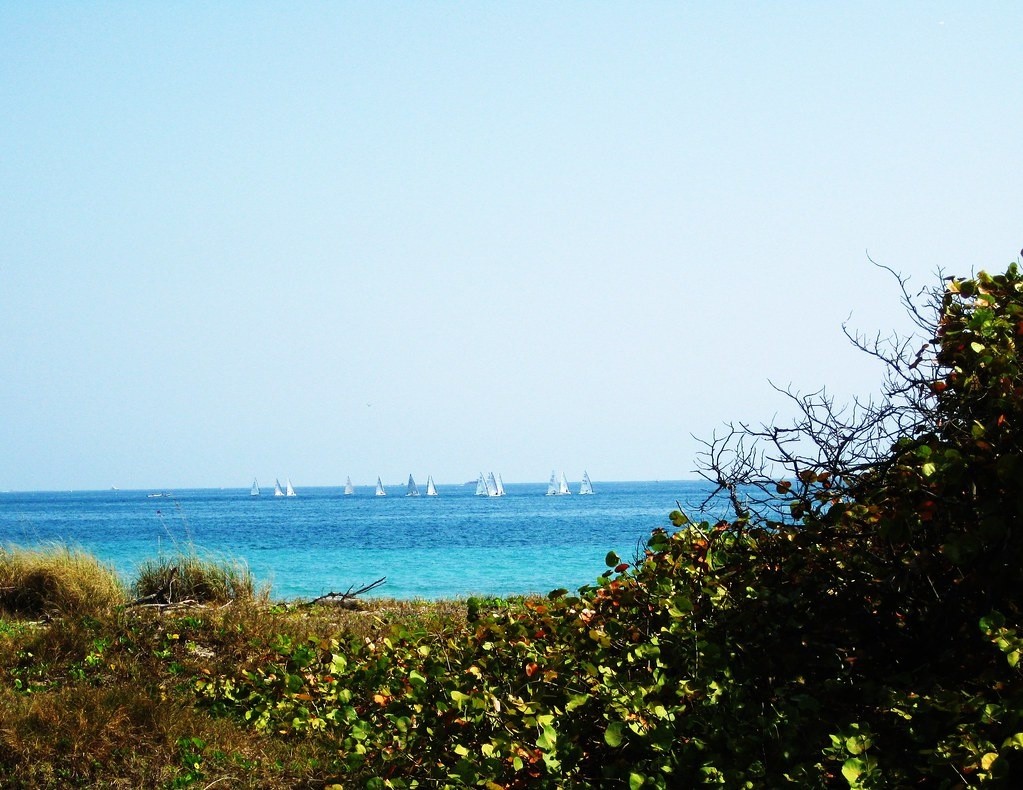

[553,472,573,495]
[284,477,297,496]
[250,477,260,496]
[425,474,439,496]
[343,475,355,496]
[546,471,557,496]
[404,474,422,496]
[375,475,387,496]
[577,469,595,495]
[273,478,286,495]
[474,471,507,497]
